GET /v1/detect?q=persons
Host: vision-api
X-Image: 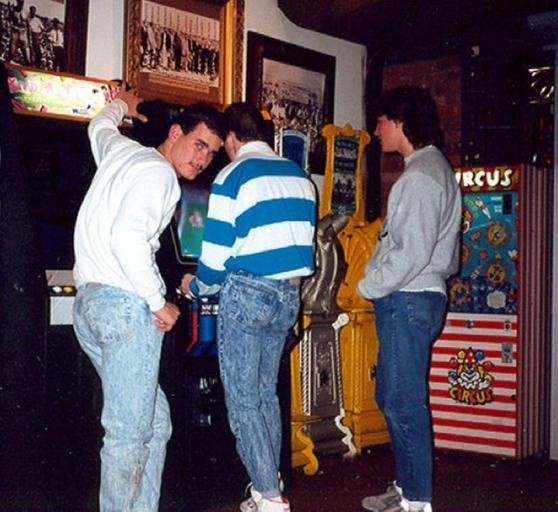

[353,81,465,512]
[332,147,356,205]
[26,5,46,68]
[7,0,30,64]
[179,101,319,512]
[262,81,320,128]
[72,80,227,511]
[36,12,64,72]
[140,22,220,77]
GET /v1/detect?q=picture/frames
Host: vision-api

[0,0,89,76]
[123,0,244,113]
[247,31,336,174]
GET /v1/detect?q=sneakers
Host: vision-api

[361,480,432,512]
[239,472,291,512]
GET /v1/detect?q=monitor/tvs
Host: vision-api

[175,183,211,257]
[35,156,96,259]
